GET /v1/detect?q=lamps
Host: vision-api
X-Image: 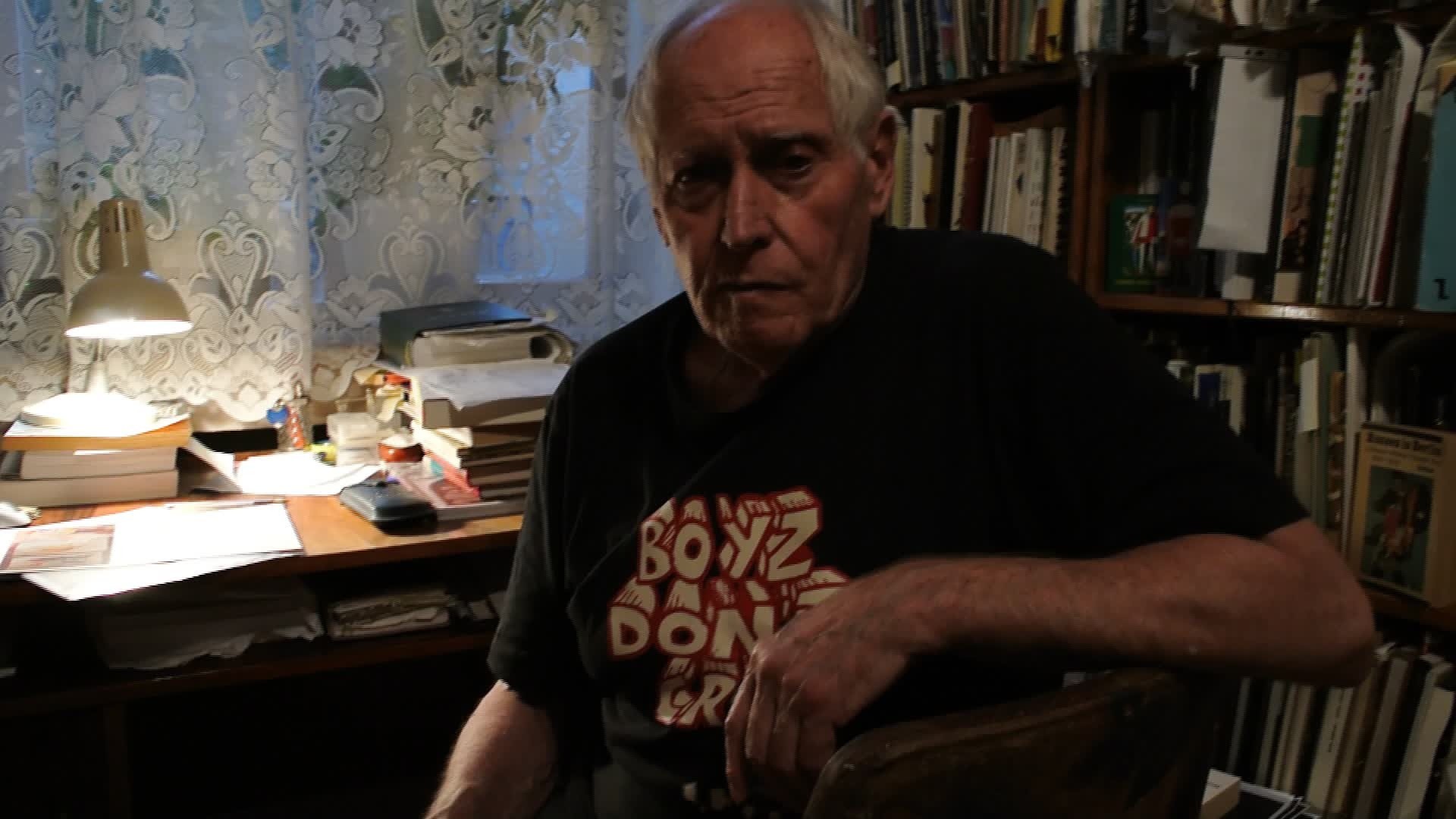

[19,194,194,425]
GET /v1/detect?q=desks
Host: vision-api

[1,433,540,819]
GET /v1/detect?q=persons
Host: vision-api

[1130,206,1157,271]
[423,0,1377,819]
[1397,488,1429,559]
[1365,480,1405,545]
[1370,492,1406,564]
[1279,219,1316,271]
[1124,213,1139,270]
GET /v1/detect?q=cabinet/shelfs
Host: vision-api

[859,1,1456,819]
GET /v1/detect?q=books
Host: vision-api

[1200,615,1456,819]
[362,301,581,523]
[846,0,1456,312]
[1149,326,1456,608]
[0,393,194,508]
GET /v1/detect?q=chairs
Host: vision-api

[802,654,1230,819]
